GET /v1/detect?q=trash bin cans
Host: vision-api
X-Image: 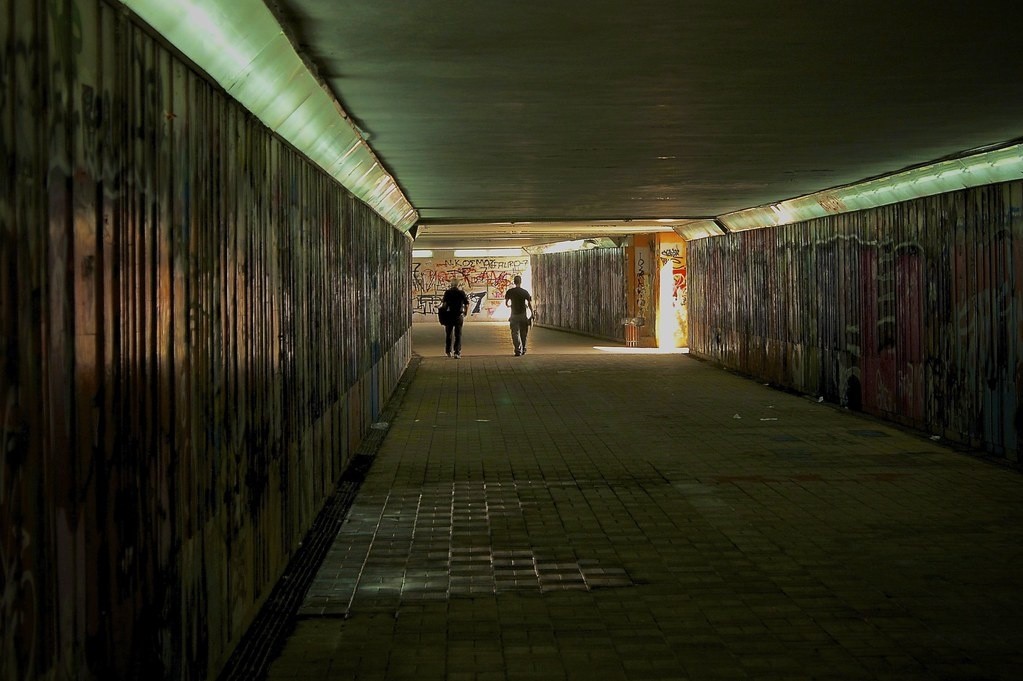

[624,323,640,348]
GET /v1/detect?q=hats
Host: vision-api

[528,317,533,330]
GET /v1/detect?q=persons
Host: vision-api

[441,278,469,359]
[505,276,534,356]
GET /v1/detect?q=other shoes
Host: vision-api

[522,348,526,355]
[514,352,520,356]
[446,352,451,357]
[454,354,461,358]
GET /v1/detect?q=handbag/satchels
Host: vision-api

[438,306,447,325]
